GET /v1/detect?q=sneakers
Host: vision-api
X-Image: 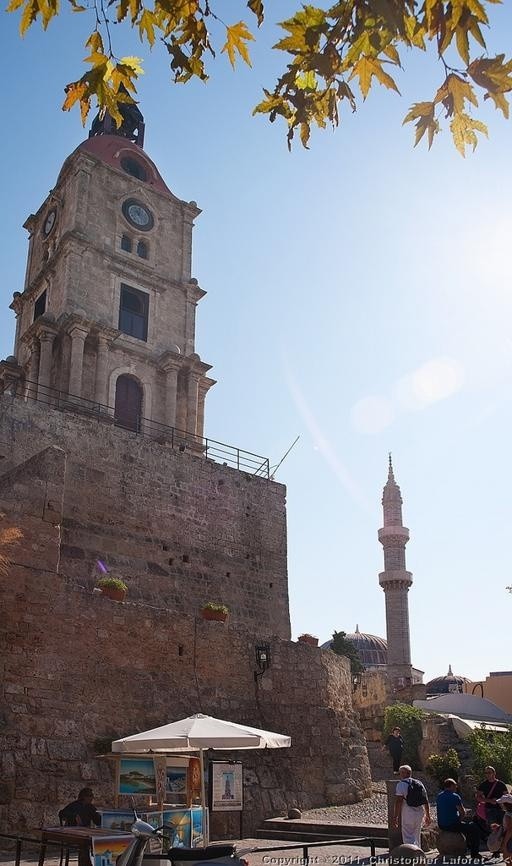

[471,854,489,862]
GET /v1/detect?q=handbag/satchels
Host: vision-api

[475,801,488,821]
[487,824,504,852]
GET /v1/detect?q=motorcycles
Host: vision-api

[116,796,249,866]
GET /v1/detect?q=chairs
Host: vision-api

[59,810,93,866]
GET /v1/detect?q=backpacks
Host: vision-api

[400,779,426,807]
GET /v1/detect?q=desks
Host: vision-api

[32,826,135,866]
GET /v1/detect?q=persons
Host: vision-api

[476,765,508,859]
[392,765,431,849]
[436,778,483,859]
[379,727,404,775]
[491,794,512,858]
[61,788,102,866]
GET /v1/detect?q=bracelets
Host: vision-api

[393,816,398,819]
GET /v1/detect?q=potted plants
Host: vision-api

[201,601,229,622]
[97,576,128,601]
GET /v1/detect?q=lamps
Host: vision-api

[254,639,271,681]
[351,671,362,694]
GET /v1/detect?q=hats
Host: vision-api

[496,794,512,804]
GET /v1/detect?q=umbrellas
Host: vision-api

[109,711,295,851]
[411,692,511,723]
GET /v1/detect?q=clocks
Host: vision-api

[44,211,56,235]
[128,203,150,226]
